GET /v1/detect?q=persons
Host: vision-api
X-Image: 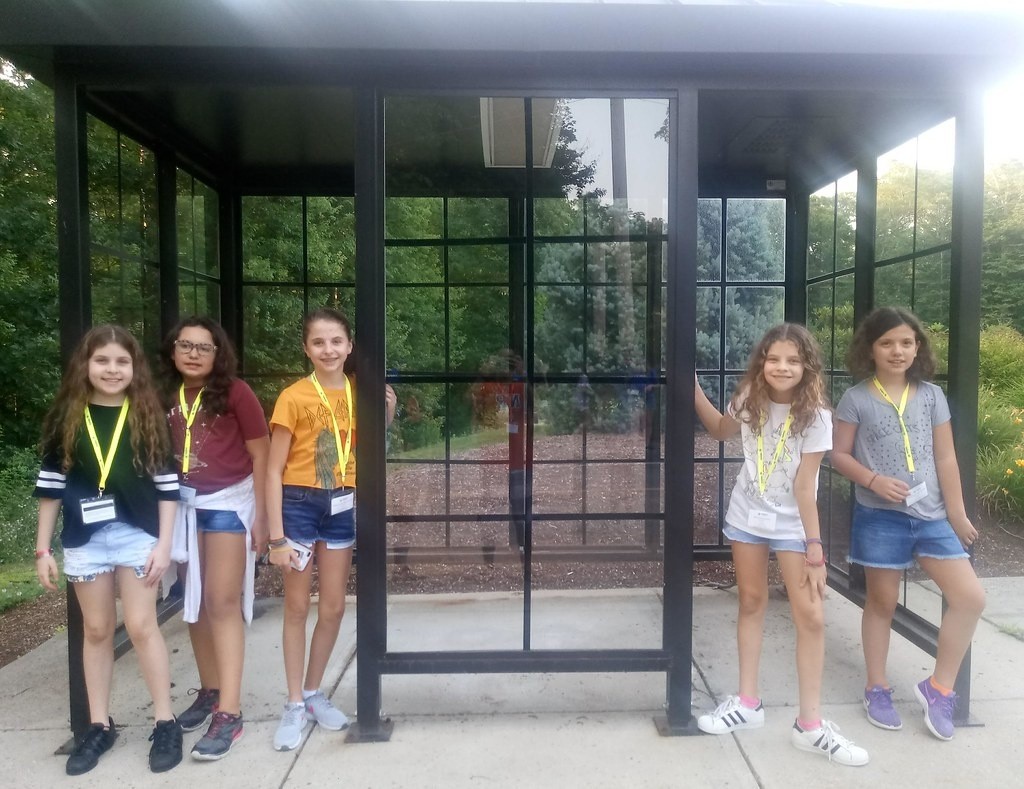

[695,322,871,767]
[33,325,182,776]
[832,305,985,740]
[159,317,271,761]
[260,308,396,751]
[462,347,548,581]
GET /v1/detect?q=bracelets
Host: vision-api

[869,473,879,487]
[268,537,293,552]
[805,538,823,548]
[804,552,826,567]
[35,549,52,559]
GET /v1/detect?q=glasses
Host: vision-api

[174,339,218,357]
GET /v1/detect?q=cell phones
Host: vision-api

[285,536,313,571]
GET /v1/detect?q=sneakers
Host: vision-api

[177,687,221,731]
[864,682,903,730]
[303,689,349,730]
[190,710,244,761]
[791,716,869,766]
[66,715,116,774]
[698,695,766,735]
[150,712,183,773]
[274,703,308,752]
[913,676,959,741]
